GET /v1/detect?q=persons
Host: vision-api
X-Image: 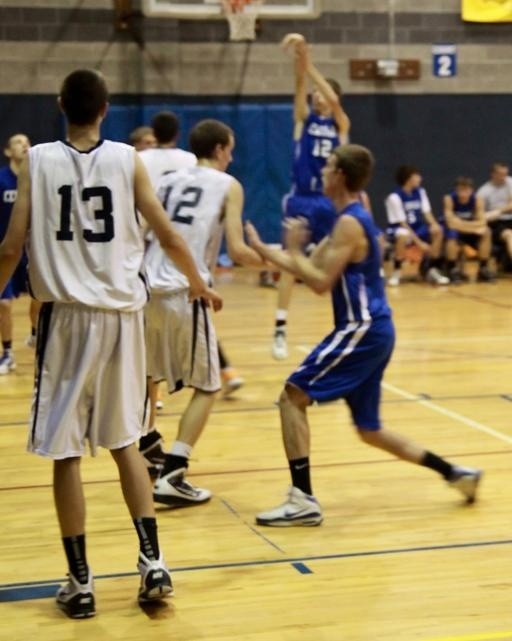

[0,132,39,376]
[243,143,483,527]
[137,120,279,505]
[443,177,495,283]
[475,161,512,277]
[271,32,351,361]
[1,68,224,619]
[385,166,451,284]
[131,111,241,410]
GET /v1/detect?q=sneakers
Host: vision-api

[152,467,211,507]
[260,272,274,287]
[272,271,281,282]
[451,467,480,504]
[139,431,170,463]
[0,349,17,374]
[272,326,287,360]
[27,336,36,348]
[388,269,499,286]
[136,553,173,601]
[57,573,96,619]
[221,368,243,394]
[256,487,323,527]
